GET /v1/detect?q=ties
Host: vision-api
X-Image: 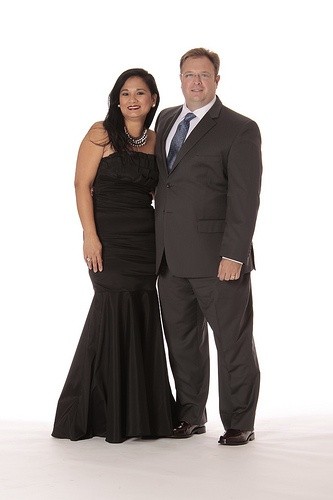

[166,112,197,174]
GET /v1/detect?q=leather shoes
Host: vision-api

[168,420,206,438]
[218,428,255,445]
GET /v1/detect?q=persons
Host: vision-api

[153,48,261,446]
[51,67,177,444]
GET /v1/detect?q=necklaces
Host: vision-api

[123,126,149,147]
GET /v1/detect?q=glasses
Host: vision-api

[181,73,215,80]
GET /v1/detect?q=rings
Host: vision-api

[231,276,235,278]
[87,258,91,261]
